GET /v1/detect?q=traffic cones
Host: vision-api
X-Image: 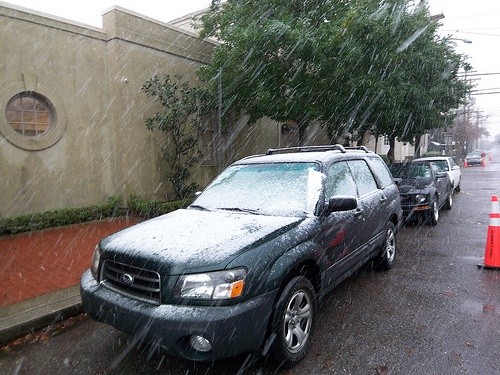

[488,153,493,161]
[464,159,468,168]
[481,157,486,167]
[477,195,500,269]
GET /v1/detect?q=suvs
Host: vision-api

[79,144,403,363]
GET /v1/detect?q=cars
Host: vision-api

[412,156,462,193]
[388,161,453,224]
[465,152,482,165]
